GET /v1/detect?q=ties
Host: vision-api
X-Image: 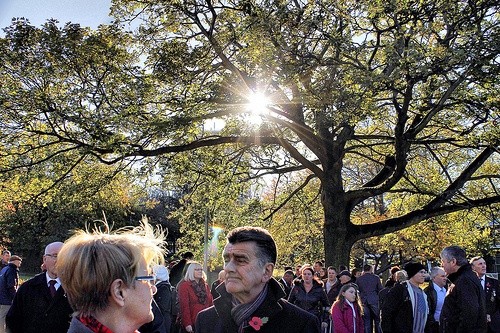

[479,279,483,290]
[49,280,58,297]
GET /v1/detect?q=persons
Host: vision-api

[287,269,329,333]
[470,256,500,333]
[299,262,328,295]
[276,265,302,299]
[381,262,428,333]
[424,266,449,333]
[356,265,382,333]
[439,246,486,333]
[195,228,321,333]
[58,227,157,333]
[169,251,194,333]
[339,266,352,290]
[178,262,214,333]
[0,251,21,333]
[351,268,360,282]
[143,265,176,333]
[6,241,74,333]
[324,267,341,304]
[331,284,364,333]
[210,270,226,300]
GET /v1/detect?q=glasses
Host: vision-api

[135,273,157,284]
[45,254,57,258]
[194,269,202,271]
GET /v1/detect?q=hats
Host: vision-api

[9,255,21,261]
[336,270,351,279]
[405,262,426,279]
